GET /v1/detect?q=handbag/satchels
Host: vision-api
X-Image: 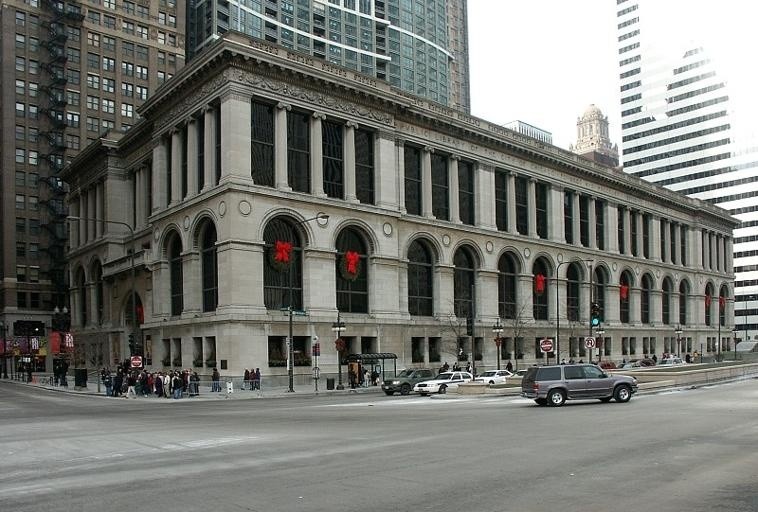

[469,366,473,371]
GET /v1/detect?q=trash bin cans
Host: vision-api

[327,377,334,390]
[75,368,87,390]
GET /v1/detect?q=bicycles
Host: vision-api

[37,374,54,385]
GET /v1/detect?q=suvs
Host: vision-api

[380,366,436,398]
[521,364,640,404]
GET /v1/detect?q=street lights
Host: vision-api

[331,311,347,390]
[54,302,69,388]
[555,257,596,363]
[310,335,321,392]
[674,324,684,360]
[746,294,754,341]
[732,328,739,359]
[0,321,11,379]
[286,212,330,393]
[492,317,506,371]
[27,327,39,381]
[65,215,140,373]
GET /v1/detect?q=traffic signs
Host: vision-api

[281,308,310,317]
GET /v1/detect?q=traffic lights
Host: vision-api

[592,302,600,324]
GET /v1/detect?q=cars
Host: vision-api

[413,369,527,396]
[590,358,688,369]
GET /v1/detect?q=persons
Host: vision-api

[101,358,200,400]
[348,365,379,389]
[645,348,699,363]
[560,357,584,365]
[241,367,260,390]
[439,361,473,373]
[209,368,222,392]
[505,359,513,372]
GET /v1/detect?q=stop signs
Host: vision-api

[130,356,141,368]
[540,340,553,352]
